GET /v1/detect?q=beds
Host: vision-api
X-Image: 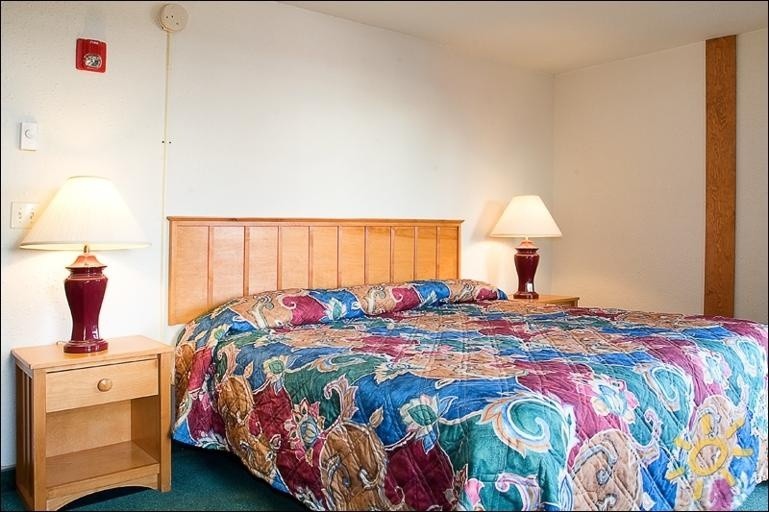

[168,215,768,511]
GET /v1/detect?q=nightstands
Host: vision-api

[15,335,174,511]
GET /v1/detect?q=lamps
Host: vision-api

[19,177,148,355]
[488,196,564,302]
